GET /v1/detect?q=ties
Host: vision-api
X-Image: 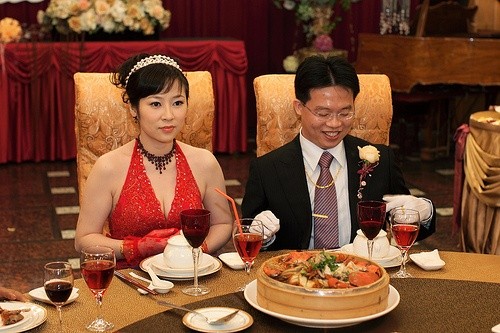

[314,152,339,249]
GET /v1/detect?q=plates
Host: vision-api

[140,253,222,281]
[342,244,403,263]
[152,255,212,272]
[1,301,48,333]
[245,278,400,328]
[29,288,79,305]
[182,307,253,333]
[379,251,411,267]
[409,253,446,270]
[219,252,254,269]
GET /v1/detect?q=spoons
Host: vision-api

[157,301,240,325]
[146,266,171,289]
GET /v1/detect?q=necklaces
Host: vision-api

[137,134,176,174]
[305,165,342,189]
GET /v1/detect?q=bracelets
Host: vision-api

[120,241,124,256]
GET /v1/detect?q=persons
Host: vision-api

[241,54,436,251]
[74,53,233,271]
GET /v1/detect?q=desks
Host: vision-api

[452,110,500,256]
[357,33,500,159]
[0,39,250,163]
[9,251,499,333]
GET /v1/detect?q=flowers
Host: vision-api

[274,0,352,72]
[35,0,172,35]
[0,18,24,45]
[354,143,383,199]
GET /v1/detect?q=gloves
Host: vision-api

[382,195,432,223]
[249,210,280,243]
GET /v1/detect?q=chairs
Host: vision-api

[253,74,394,157]
[72,70,214,237]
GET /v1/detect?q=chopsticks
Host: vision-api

[114,269,158,295]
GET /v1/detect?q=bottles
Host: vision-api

[163,235,202,268]
[353,229,389,257]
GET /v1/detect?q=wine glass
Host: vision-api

[80,245,116,332]
[179,209,211,296]
[358,200,386,261]
[232,218,264,292]
[44,261,74,333]
[391,209,420,278]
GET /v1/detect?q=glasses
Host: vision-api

[301,102,355,120]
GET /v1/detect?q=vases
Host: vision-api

[295,47,348,66]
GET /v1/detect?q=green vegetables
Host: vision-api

[299,248,352,280]
